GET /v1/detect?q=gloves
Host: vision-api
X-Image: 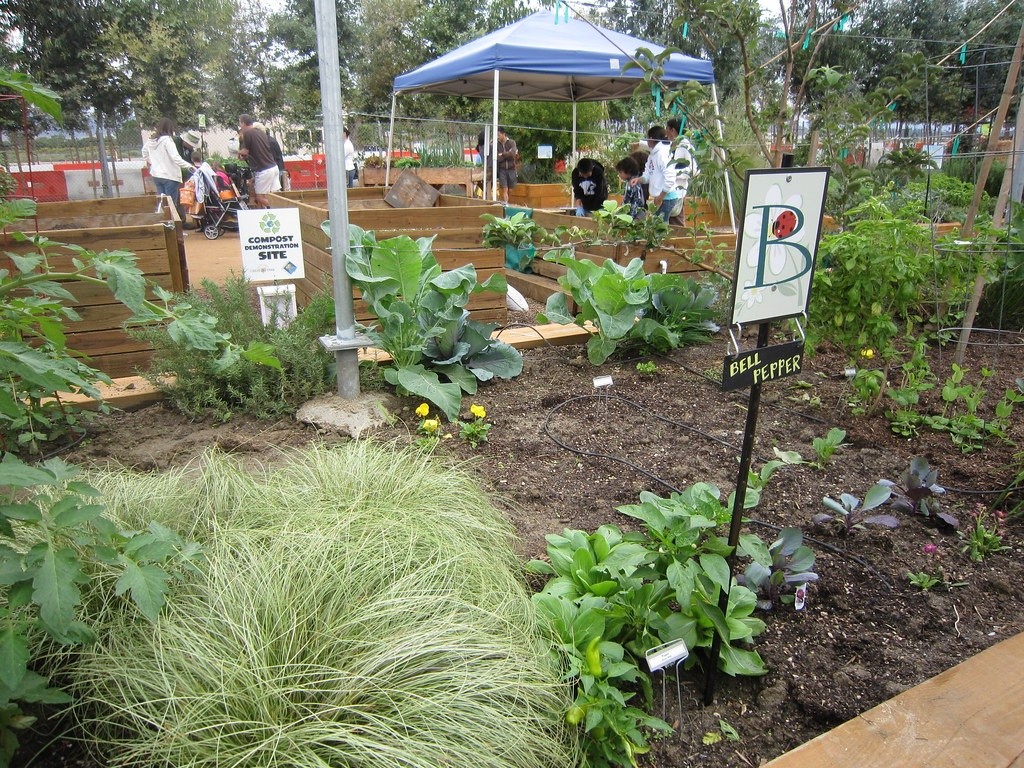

[576,206,585,216]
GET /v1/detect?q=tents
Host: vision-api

[386,9,736,235]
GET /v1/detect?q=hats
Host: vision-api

[252,122,267,133]
[180,129,202,148]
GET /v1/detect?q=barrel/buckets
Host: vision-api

[179,182,195,205]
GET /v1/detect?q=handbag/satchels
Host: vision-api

[670,194,683,217]
[353,163,359,179]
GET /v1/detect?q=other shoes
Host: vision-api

[502,202,508,207]
[183,232,188,237]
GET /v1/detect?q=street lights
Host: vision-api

[0,93,39,237]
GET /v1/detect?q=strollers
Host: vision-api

[197,162,253,241]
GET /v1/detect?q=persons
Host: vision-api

[173,131,201,230]
[571,158,608,218]
[211,161,232,194]
[630,126,681,225]
[616,151,650,221]
[476,126,517,203]
[141,118,197,236]
[191,152,203,169]
[343,126,356,188]
[237,115,291,209]
[666,117,701,227]
